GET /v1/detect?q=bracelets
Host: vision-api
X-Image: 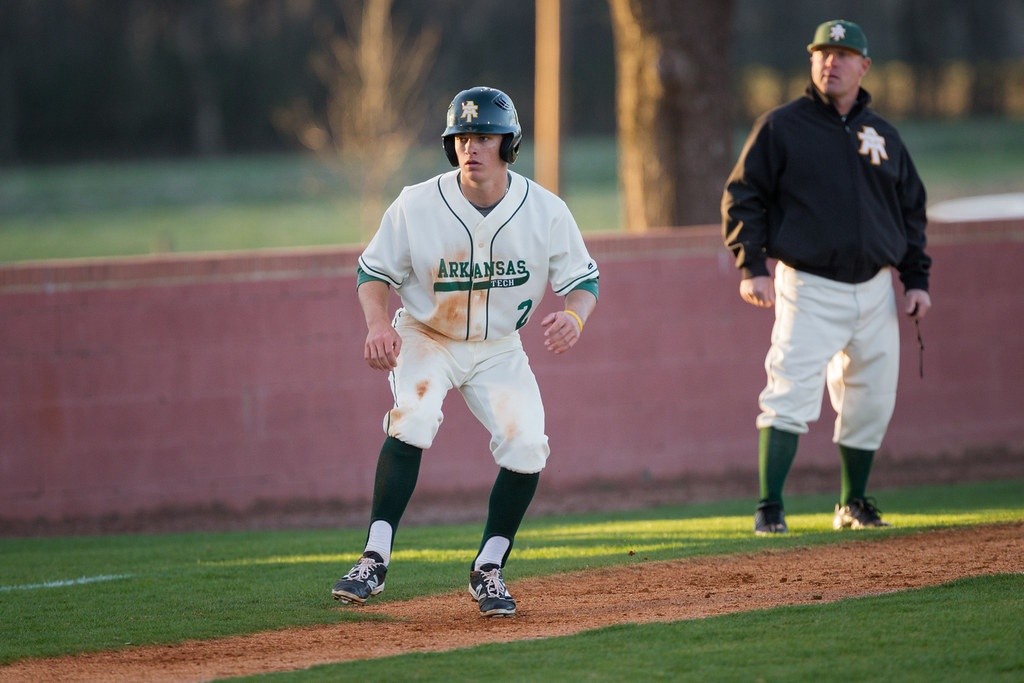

[563,309,583,333]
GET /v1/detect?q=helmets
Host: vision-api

[440,86,523,167]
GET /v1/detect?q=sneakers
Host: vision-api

[331,550,388,604]
[833,496,892,531]
[753,500,788,536]
[468,563,517,617]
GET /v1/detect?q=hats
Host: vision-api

[806,18,868,58]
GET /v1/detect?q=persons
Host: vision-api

[719,19,934,537]
[330,87,601,620]
